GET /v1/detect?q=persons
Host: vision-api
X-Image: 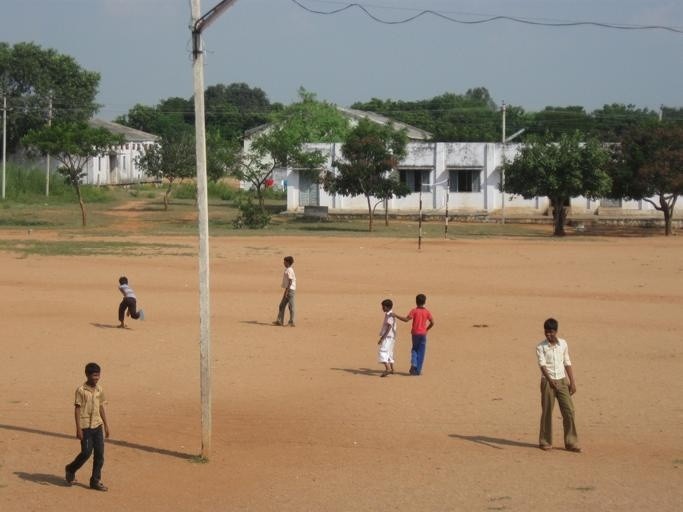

[385,293,435,376]
[64,362,111,491]
[116,275,145,330]
[377,299,397,378]
[534,317,583,453]
[271,255,297,327]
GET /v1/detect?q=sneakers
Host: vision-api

[65,464,74,485]
[572,447,583,452]
[139,308,144,321]
[380,370,390,377]
[409,365,420,375]
[90,477,108,491]
[272,321,295,327]
[542,444,552,450]
